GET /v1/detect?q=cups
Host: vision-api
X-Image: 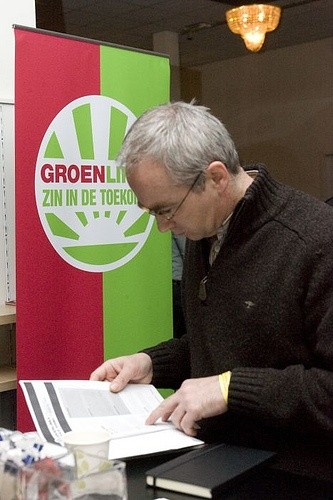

[63,429,112,477]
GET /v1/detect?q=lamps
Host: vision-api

[226,4,281,53]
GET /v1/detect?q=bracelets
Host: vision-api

[219,370,231,404]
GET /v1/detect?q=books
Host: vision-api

[146,442,275,498]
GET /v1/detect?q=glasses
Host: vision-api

[138,170,202,219]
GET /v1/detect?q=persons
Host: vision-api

[90,101,333,480]
